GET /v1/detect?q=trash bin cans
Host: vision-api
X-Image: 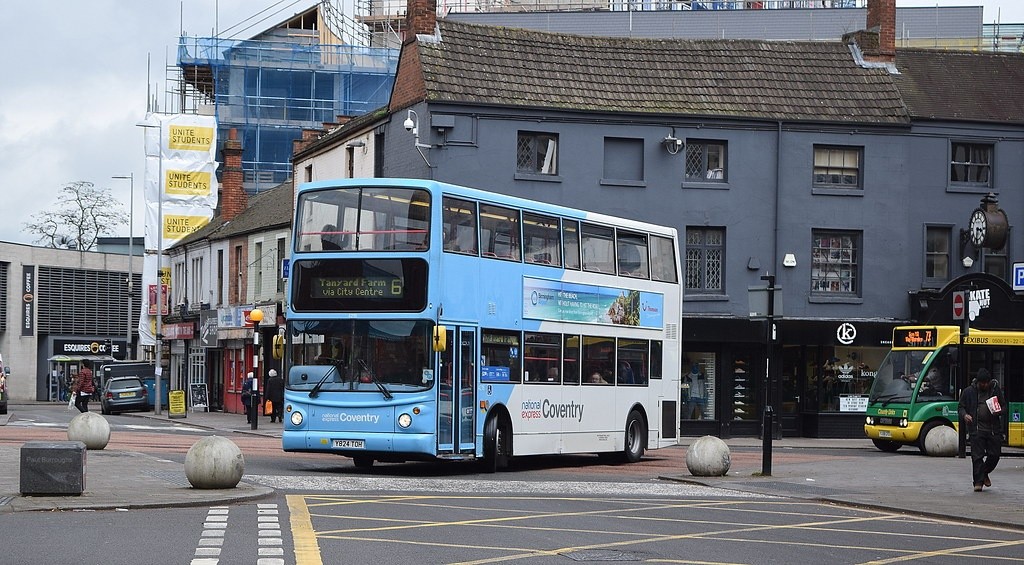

[143,377,167,409]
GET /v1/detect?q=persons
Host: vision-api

[421,227,462,252]
[686,362,708,419]
[548,351,628,384]
[59,359,94,412]
[241,372,261,430]
[321,224,349,250]
[314,342,348,369]
[958,367,1008,491]
[266,369,283,422]
[901,360,942,396]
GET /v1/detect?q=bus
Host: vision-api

[100,360,170,410]
[865,325,1024,457]
[272,177,681,473]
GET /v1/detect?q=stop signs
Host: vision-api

[953,292,966,320]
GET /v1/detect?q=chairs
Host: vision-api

[367,244,674,282]
[525,361,647,385]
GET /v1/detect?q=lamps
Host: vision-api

[346,141,365,147]
[661,134,678,146]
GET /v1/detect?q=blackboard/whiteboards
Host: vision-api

[189,383,209,407]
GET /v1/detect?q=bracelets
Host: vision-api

[915,377,917,382]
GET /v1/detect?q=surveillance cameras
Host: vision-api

[404,119,414,131]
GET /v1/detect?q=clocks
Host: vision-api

[970,211,986,245]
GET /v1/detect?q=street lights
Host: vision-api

[111,173,133,360]
[250,308,264,430]
[136,123,162,414]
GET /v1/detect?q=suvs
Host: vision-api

[98,375,148,415]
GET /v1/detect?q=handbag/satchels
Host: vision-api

[243,382,251,405]
[264,399,272,414]
[68,393,76,410]
[84,386,94,392]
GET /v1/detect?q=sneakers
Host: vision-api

[983,474,992,486]
[974,482,982,491]
